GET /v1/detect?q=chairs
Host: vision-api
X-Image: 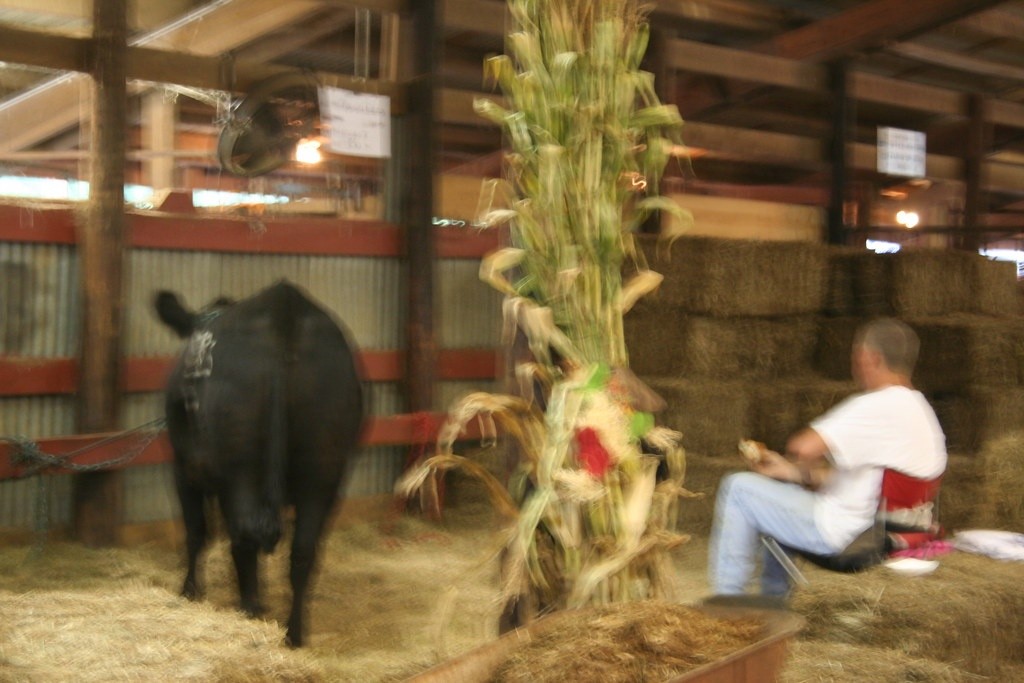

[758,468,946,616]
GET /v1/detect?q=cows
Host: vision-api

[152,275,369,651]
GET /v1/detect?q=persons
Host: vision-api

[705,317,948,601]
